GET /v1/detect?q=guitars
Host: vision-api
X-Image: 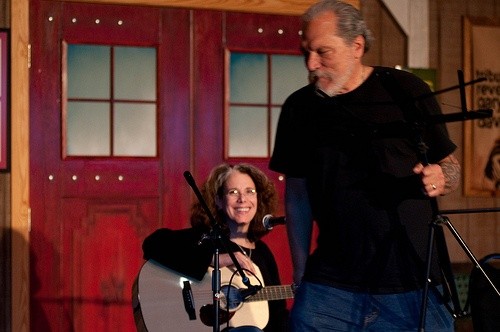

[131,258,294,332]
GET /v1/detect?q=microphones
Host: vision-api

[261,213,288,231]
[235,285,262,301]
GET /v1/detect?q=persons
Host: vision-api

[267,0,461,332]
[141,163,290,323]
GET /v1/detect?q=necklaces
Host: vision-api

[229,238,252,260]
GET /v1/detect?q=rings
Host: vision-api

[432,184,437,190]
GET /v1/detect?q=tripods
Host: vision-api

[417,128,500,332]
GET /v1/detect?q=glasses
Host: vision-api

[223,188,257,196]
